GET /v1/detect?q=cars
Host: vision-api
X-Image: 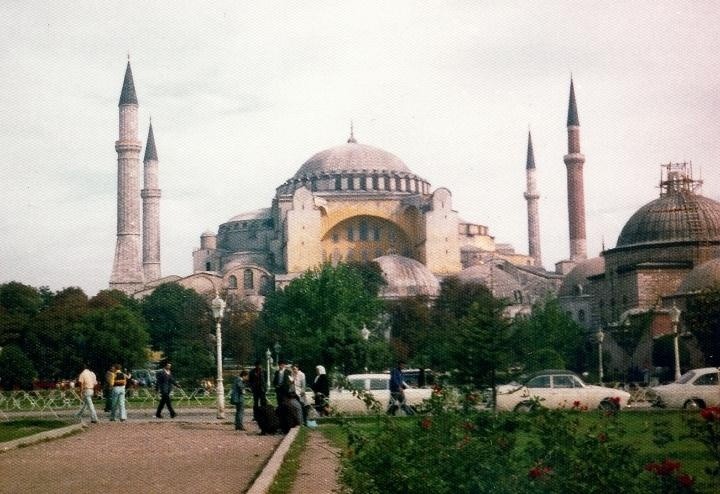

[127,369,163,386]
[644,367,720,410]
[483,370,630,414]
[304,369,440,416]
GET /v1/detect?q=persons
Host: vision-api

[229,358,331,436]
[154,362,181,418]
[102,364,139,422]
[73,362,99,423]
[642,366,648,385]
[386,360,414,417]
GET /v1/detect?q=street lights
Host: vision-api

[670,301,681,380]
[359,325,371,372]
[266,348,271,395]
[212,296,226,418]
[274,342,281,368]
[597,328,604,379]
